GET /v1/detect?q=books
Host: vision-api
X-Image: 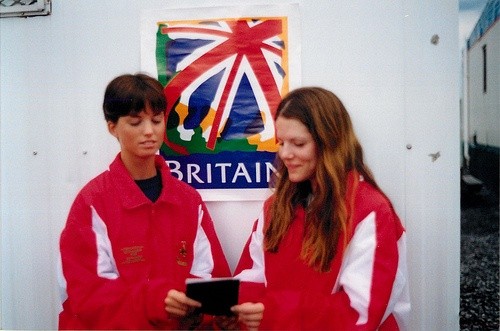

[184,276,240,319]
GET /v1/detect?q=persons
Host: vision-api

[54,72,231,329]
[227,86,411,330]
